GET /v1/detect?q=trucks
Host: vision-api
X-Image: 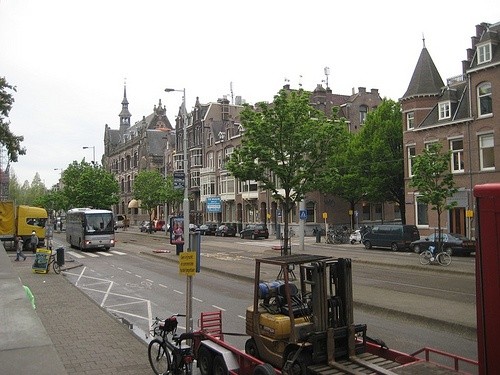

[0,202,48,251]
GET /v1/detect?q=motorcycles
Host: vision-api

[349,229,362,246]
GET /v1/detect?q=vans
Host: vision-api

[118,215,129,229]
[363,226,419,253]
[216,224,236,237]
[239,225,269,241]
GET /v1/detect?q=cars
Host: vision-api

[148,218,167,229]
[409,234,474,255]
[189,223,201,231]
[140,221,151,232]
[197,224,217,235]
[162,224,171,231]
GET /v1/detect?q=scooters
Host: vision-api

[147,311,194,374]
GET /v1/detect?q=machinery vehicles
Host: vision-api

[245,248,479,375]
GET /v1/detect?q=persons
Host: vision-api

[14,236,27,261]
[30,231,39,254]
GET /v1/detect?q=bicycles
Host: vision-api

[287,228,295,237]
[49,251,64,273]
[311,225,361,245]
[418,240,452,267]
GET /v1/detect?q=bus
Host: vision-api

[65,208,116,251]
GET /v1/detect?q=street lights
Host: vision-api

[54,168,62,177]
[82,146,95,165]
[163,87,186,114]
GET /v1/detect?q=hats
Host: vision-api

[33,232,37,234]
